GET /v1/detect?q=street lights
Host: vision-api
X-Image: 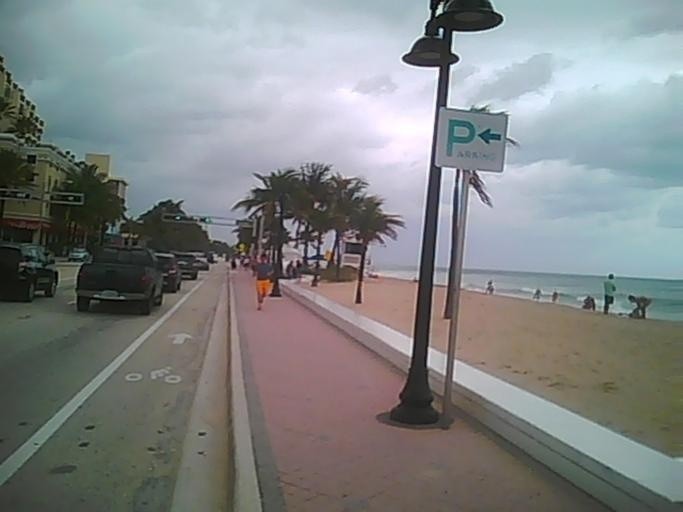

[268,193,289,297]
[373,0,504,433]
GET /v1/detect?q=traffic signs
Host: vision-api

[0,190,31,200]
[49,192,84,204]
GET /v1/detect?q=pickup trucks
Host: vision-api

[74,243,165,316]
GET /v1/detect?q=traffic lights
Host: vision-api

[197,217,212,224]
[163,213,185,223]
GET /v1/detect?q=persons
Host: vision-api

[532,288,542,300]
[229,254,303,281]
[627,295,650,320]
[486,281,494,294]
[582,297,596,311]
[603,275,616,313]
[252,254,274,310]
[552,292,559,302]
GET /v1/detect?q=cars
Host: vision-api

[304,253,330,269]
[0,240,62,304]
[67,242,219,294]
